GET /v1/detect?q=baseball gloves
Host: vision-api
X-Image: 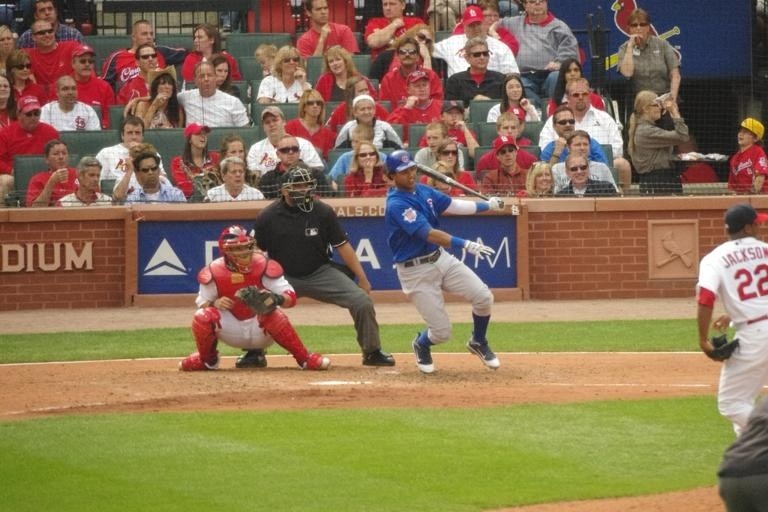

[238,287,283,314]
[707,336,738,361]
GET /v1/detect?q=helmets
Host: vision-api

[387,150,417,173]
[219,225,257,273]
[281,167,318,214]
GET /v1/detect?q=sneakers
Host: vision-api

[178,353,220,371]
[466,336,501,369]
[235,351,267,367]
[412,332,435,373]
[360,351,396,366]
[298,353,330,370]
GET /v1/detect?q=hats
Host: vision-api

[462,6,485,26]
[740,117,765,141]
[724,204,768,236]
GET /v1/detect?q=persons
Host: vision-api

[385,151,504,374]
[178,222,331,371]
[234,165,395,368]
[717,394,768,512]
[695,204,767,436]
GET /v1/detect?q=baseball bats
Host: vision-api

[418,164,505,209]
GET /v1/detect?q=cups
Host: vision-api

[59,169,69,183]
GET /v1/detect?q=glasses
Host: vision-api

[12,21,663,174]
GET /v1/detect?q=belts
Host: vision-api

[400,249,441,268]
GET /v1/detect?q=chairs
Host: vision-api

[14,32,622,207]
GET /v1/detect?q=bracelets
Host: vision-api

[209,301,214,306]
[451,237,465,250]
[476,201,489,213]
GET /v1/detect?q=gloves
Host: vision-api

[464,239,496,260]
[489,197,506,212]
